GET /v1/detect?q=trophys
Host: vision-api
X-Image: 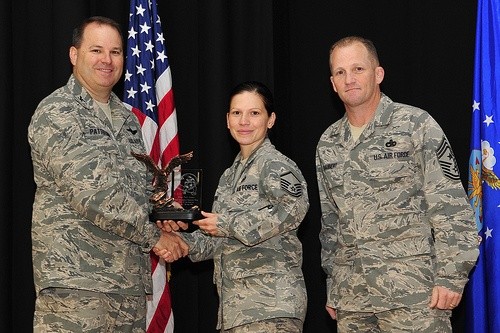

[132,151,203,221]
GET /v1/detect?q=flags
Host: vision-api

[123,0,182,333]
[468,0,500,333]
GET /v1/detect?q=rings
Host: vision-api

[450,304,456,308]
[205,231,209,234]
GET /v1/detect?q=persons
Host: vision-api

[316,37,480,333]
[152,80,309,333]
[28,16,190,333]
